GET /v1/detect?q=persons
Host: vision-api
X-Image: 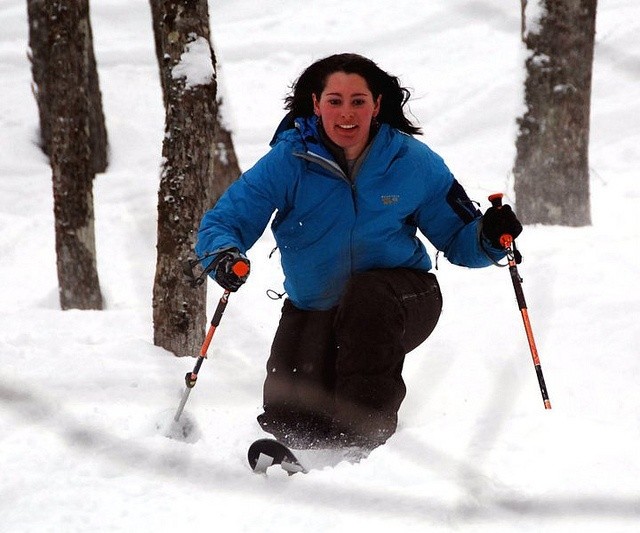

[195,52,523,449]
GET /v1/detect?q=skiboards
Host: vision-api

[246,438,383,477]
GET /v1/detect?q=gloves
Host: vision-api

[215,251,250,292]
[483,204,522,249]
